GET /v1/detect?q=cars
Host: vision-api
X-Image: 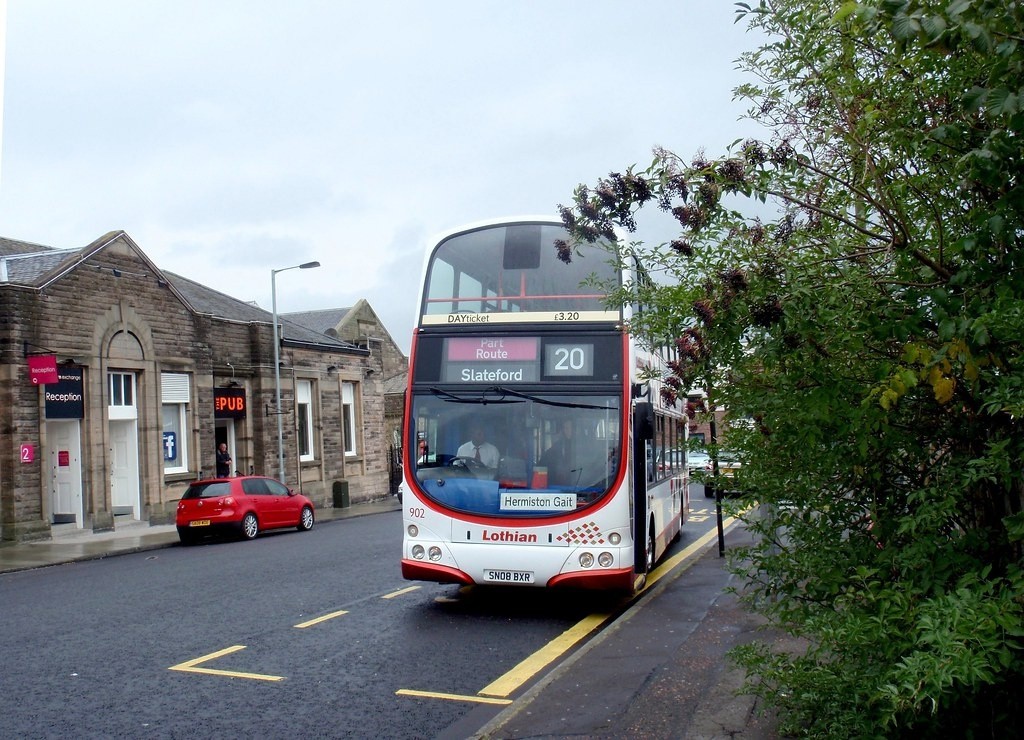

[689,451,710,475]
[176,475,316,546]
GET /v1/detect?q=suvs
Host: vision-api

[704,449,751,497]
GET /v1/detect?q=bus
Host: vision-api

[402,214,689,597]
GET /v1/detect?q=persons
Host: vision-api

[456,422,500,470]
[540,420,582,487]
[216,443,231,478]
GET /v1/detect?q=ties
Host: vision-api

[475,447,481,462]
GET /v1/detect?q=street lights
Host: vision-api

[271,260,321,483]
